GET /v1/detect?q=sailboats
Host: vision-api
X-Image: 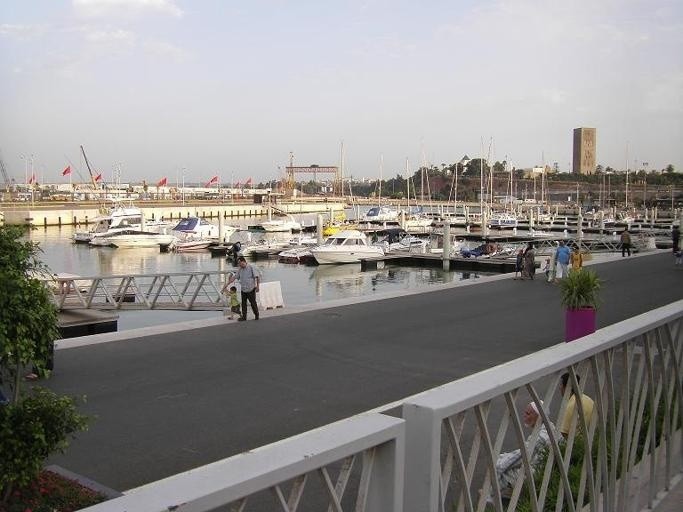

[347,136,555,226]
[569,159,635,226]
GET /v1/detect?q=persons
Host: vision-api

[620,226,630,258]
[513,237,583,283]
[224,256,260,322]
[222,286,242,320]
[673,247,682,266]
[559,371,595,438]
[476,400,566,507]
[671,224,678,255]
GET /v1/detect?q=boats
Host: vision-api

[429,234,466,253]
[372,229,428,252]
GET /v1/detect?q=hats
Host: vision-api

[530,400,544,415]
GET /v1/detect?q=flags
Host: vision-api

[156,178,166,187]
[62,166,70,176]
[28,174,35,184]
[204,181,210,187]
[210,176,217,183]
[246,178,251,184]
[94,174,100,181]
[235,182,239,187]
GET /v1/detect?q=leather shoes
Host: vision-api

[254,313,259,320]
[238,318,246,321]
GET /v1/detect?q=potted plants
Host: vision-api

[557,265,606,344]
